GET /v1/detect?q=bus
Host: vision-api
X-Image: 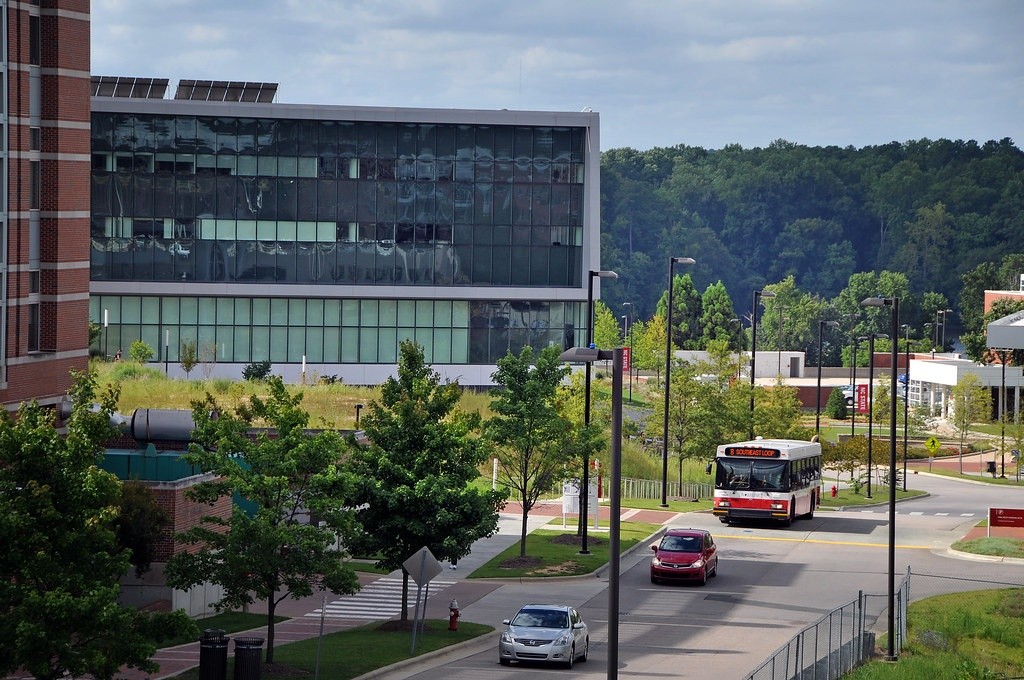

[705,434,822,528]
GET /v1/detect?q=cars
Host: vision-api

[498,604,589,669]
[899,373,909,383]
[650,528,719,585]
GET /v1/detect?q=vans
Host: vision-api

[843,386,876,405]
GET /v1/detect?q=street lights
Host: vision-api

[561,345,623,679]
[852,337,867,437]
[903,340,922,492]
[816,320,841,443]
[660,257,695,507]
[902,309,953,351]
[581,271,617,553]
[620,301,633,403]
[861,298,898,661]
[995,350,1011,477]
[731,318,741,380]
[751,290,775,440]
[773,304,790,377]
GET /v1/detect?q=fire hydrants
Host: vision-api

[448,597,461,629]
[831,487,836,498]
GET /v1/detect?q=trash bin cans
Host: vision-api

[199,628,231,680]
[987,462,996,473]
[233,637,265,680]
